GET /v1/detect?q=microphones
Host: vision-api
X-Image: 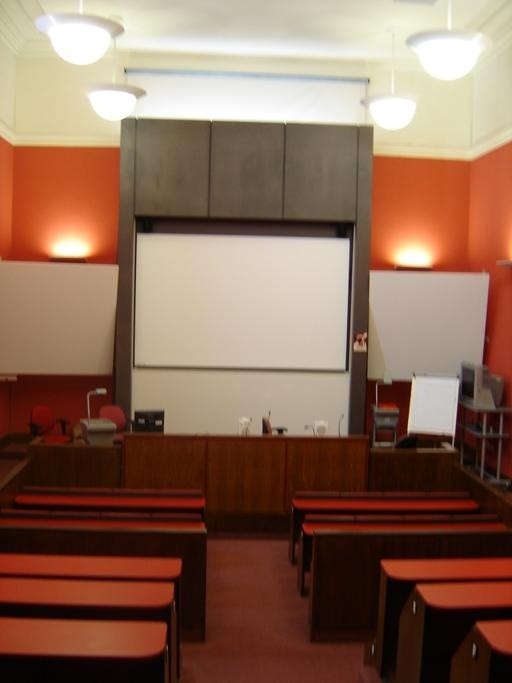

[336,411,346,436]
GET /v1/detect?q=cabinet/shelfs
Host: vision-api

[458,403,511,488]
[136,117,361,228]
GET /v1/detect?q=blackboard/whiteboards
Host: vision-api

[407,375,460,437]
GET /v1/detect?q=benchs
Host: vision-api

[288,490,512,683]
[1,486,205,680]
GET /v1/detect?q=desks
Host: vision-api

[28,435,122,486]
[369,440,457,493]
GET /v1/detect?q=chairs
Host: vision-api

[97,404,134,443]
[28,404,72,444]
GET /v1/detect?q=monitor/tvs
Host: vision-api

[134,410,165,434]
[459,359,504,409]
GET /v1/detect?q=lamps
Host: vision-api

[34,0,147,120]
[361,0,486,132]
